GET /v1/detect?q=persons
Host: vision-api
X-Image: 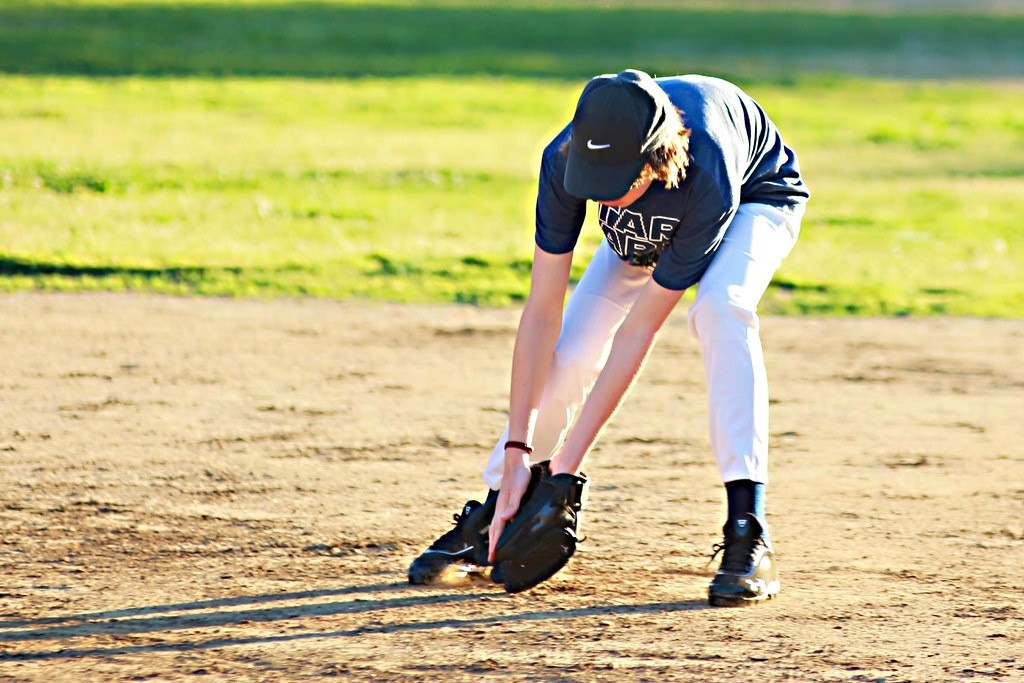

[407,68,811,607]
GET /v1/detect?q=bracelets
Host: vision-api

[503,441,532,454]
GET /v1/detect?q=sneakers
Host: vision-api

[707,512,780,607]
[409,490,506,586]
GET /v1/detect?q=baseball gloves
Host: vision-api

[488,460,589,594]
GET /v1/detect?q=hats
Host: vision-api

[563,69,670,199]
